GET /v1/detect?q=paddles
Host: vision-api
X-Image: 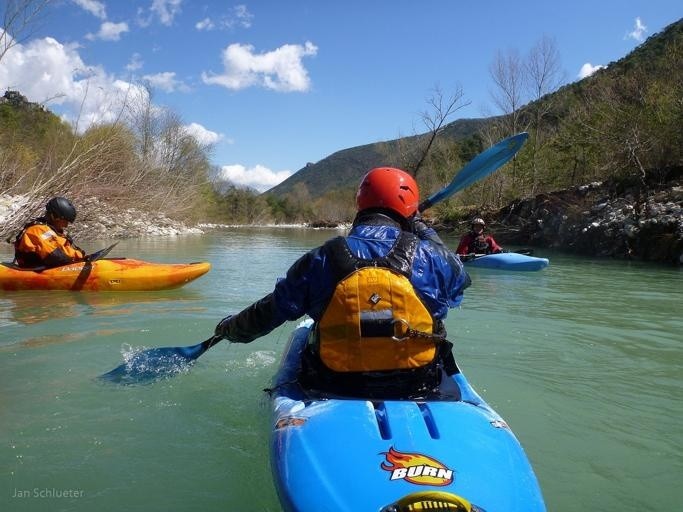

[457,248,532,260]
[80,243,118,261]
[92,132,530,384]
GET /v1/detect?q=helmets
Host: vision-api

[470,218,486,225]
[356,166,420,219]
[44,196,77,224]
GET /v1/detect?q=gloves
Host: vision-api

[214,314,234,342]
[411,219,428,232]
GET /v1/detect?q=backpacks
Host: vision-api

[295,228,460,401]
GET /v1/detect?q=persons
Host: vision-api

[15,197,91,271]
[217,167,472,399]
[455,218,504,262]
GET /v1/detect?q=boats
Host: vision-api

[457,252,550,273]
[263,317,548,512]
[1,257,212,293]
